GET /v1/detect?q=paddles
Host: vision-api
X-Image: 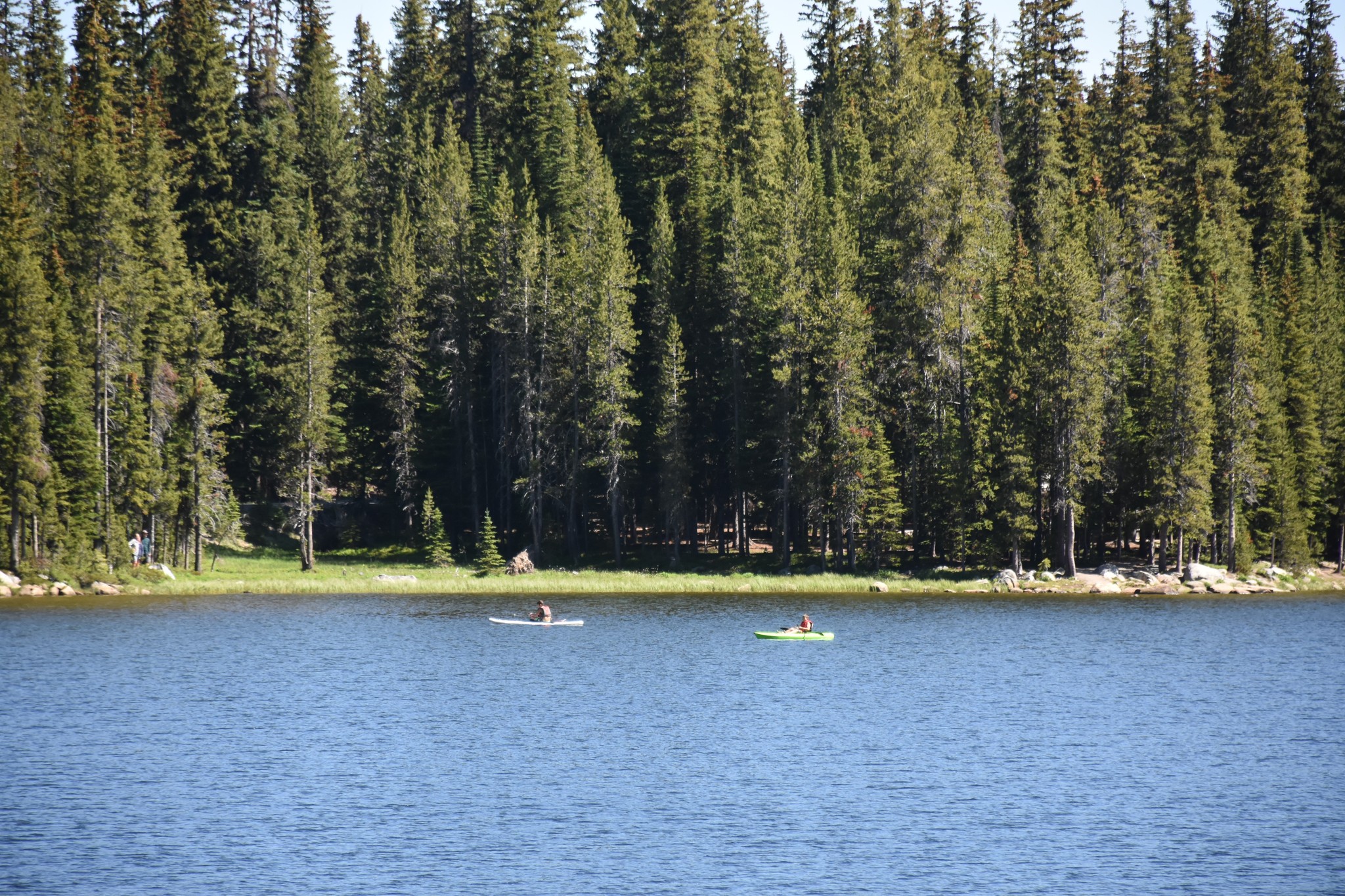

[780,627,824,636]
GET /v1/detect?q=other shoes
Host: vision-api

[783,630,788,634]
[776,630,782,633]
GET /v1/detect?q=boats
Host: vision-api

[753,631,834,641]
[487,617,584,626]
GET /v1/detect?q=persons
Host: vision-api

[776,614,812,634]
[529,600,551,623]
[141,530,152,566]
[128,533,144,569]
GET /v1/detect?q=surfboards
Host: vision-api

[489,618,583,626]
